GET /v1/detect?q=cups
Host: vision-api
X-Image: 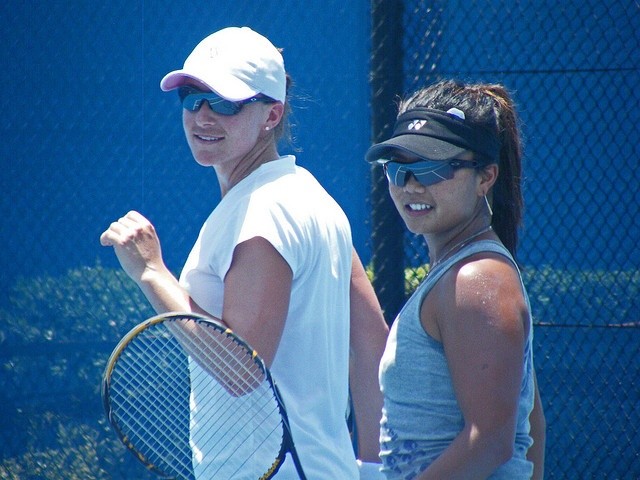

[177,79,273,116]
[383,161,482,186]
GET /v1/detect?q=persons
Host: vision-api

[99,27,390,479]
[366,78,547,479]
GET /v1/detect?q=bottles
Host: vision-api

[160,26,286,103]
[365,109,502,162]
